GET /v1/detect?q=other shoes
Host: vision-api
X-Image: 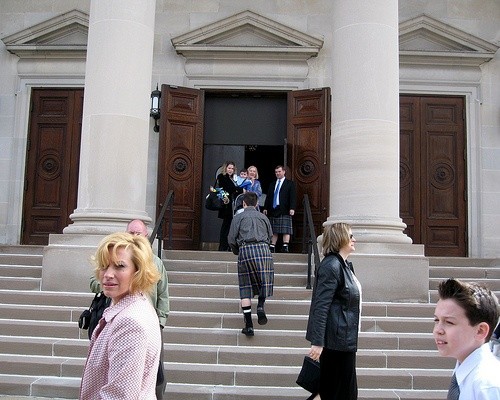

[257,307,268,325]
[242,328,254,336]
[282,242,289,252]
[269,244,276,252]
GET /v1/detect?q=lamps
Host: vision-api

[150,82,161,132]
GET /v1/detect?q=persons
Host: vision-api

[79,231,163,399]
[263,164,297,252]
[306,224,362,400]
[433,277,500,400]
[236,168,252,193]
[90,219,170,400]
[227,192,274,336]
[243,165,262,212]
[217,160,237,252]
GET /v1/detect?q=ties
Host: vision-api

[273,179,281,208]
[447,373,460,400]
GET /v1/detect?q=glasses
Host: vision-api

[350,235,353,239]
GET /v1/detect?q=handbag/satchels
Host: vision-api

[88,296,107,341]
[78,310,90,330]
[204,178,224,211]
[295,355,321,395]
